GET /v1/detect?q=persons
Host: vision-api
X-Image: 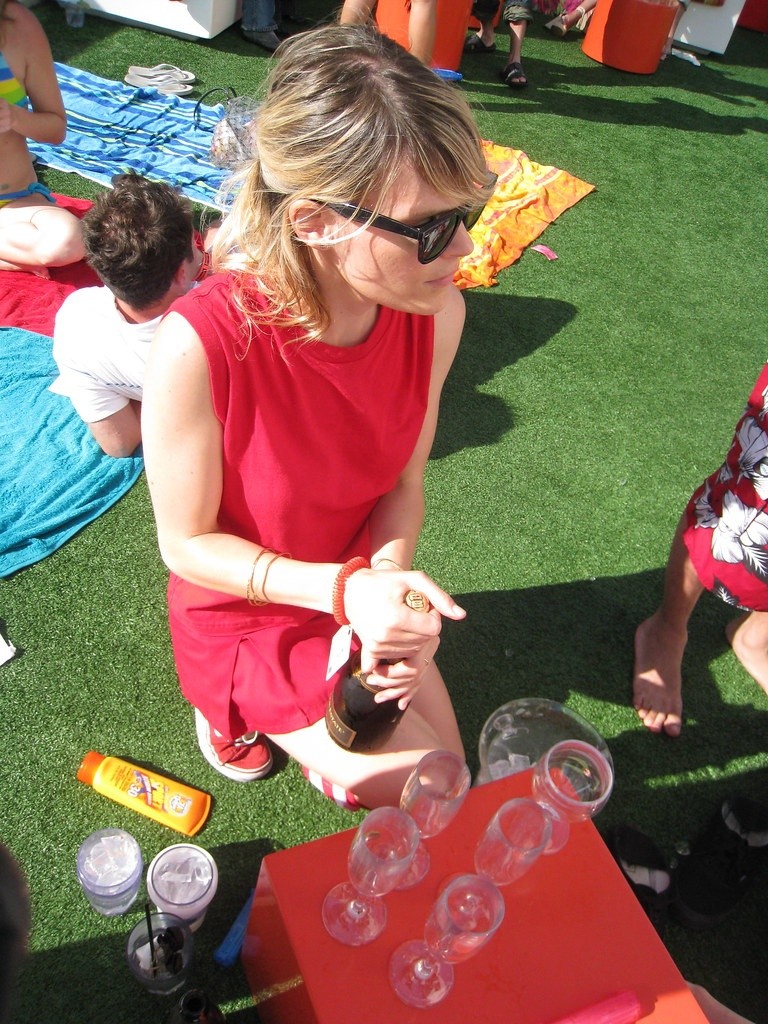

[51,172,262,458]
[0,0,91,280]
[337,0,696,90]
[632,363,768,736]
[139,27,498,809]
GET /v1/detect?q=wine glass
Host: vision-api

[424,797,551,964]
[531,739,613,860]
[320,806,417,944]
[389,871,505,1007]
[391,751,472,893]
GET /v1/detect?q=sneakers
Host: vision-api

[600,821,671,938]
[194,706,274,782]
[669,795,768,930]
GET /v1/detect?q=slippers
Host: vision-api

[128,63,196,85]
[124,73,193,96]
[464,32,496,53]
[502,63,528,88]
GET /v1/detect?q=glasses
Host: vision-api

[311,168,499,267]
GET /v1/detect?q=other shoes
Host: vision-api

[244,28,291,52]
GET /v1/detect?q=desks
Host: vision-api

[242,775,709,1024]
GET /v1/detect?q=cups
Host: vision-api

[147,842,219,935]
[126,912,191,994]
[75,829,142,918]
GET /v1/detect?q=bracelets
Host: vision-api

[372,558,402,569]
[331,556,370,626]
[246,548,292,607]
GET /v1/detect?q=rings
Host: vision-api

[424,658,429,663]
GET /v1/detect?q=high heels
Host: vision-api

[543,12,568,36]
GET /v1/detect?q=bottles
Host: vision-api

[326,590,433,753]
